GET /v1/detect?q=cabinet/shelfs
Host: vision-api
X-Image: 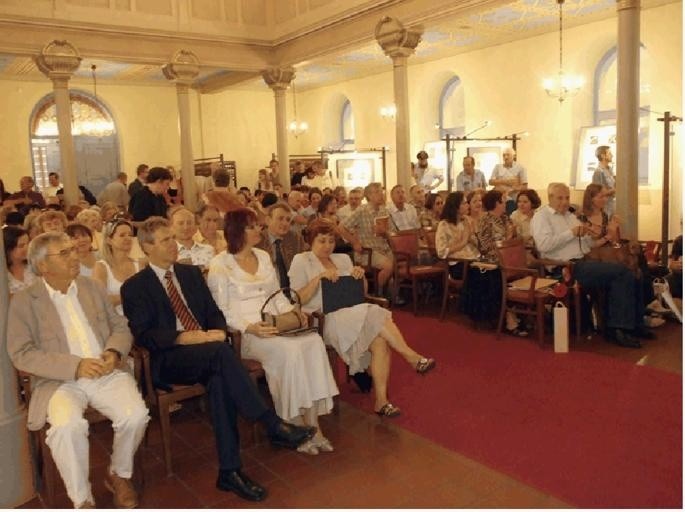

[194,154,237,190]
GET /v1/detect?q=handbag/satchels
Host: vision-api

[470,261,498,273]
[321,277,365,315]
[577,226,634,271]
[261,287,307,337]
[554,303,568,353]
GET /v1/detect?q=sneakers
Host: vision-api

[386,294,405,306]
[372,293,383,299]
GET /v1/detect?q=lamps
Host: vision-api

[70,64,117,142]
[542,1,588,103]
[289,79,309,139]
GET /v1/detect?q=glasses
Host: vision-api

[45,247,78,256]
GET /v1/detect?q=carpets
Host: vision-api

[338,307,682,509]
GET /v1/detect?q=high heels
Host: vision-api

[643,315,666,328]
[315,438,334,452]
[416,357,436,377]
[374,404,401,421]
[646,300,672,314]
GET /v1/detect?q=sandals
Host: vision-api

[514,318,534,330]
[504,324,529,337]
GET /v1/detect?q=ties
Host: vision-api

[25,192,29,199]
[275,239,290,299]
[164,272,199,331]
[64,296,91,358]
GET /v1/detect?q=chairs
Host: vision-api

[21,351,143,509]
[352,246,387,297]
[389,233,446,317]
[493,236,581,348]
[426,231,476,323]
[313,295,389,415]
[227,313,313,448]
[635,239,673,279]
[134,324,238,477]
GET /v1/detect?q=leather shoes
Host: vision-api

[103,472,139,508]
[613,328,640,347]
[631,327,657,340]
[216,470,268,502]
[270,424,317,450]
[297,442,319,455]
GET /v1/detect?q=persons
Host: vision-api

[53,184,96,207]
[172,207,215,269]
[90,218,145,317]
[478,190,514,250]
[456,157,487,192]
[5,231,151,509]
[666,220,683,317]
[201,169,243,228]
[255,204,306,299]
[249,190,264,210]
[313,162,336,190]
[2,225,34,298]
[256,169,273,192]
[113,211,146,259]
[385,185,430,266]
[19,203,41,230]
[270,160,280,185]
[121,217,318,502]
[176,168,185,205]
[240,187,250,196]
[290,160,306,187]
[5,212,25,228]
[128,164,149,197]
[466,189,485,221]
[41,172,63,205]
[287,190,317,233]
[75,209,103,252]
[291,223,436,422]
[0,180,11,207]
[301,168,315,185]
[163,166,177,206]
[592,145,616,218]
[299,186,311,207]
[435,194,532,335]
[47,204,60,211]
[236,191,263,214]
[261,193,288,214]
[308,195,337,225]
[489,148,528,212]
[204,207,340,456]
[408,186,425,213]
[420,195,443,244]
[100,201,118,234]
[576,185,670,328]
[101,172,131,217]
[37,210,68,235]
[333,186,348,208]
[299,188,323,249]
[410,163,416,186]
[193,206,227,254]
[334,189,360,243]
[337,182,404,305]
[413,151,443,193]
[129,167,173,237]
[530,185,656,349]
[508,189,540,248]
[66,225,101,277]
[272,183,282,198]
